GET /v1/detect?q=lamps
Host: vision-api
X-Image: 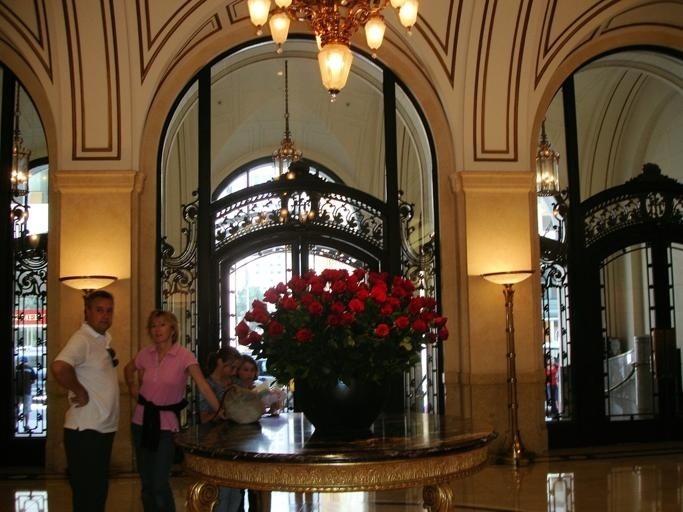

[480,270,535,467]
[271,60,302,161]
[10,82,31,198]
[246,0,418,99]
[534,119,560,197]
[58,275,117,321]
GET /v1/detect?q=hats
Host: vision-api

[21,356,28,362]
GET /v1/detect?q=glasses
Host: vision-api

[107,347,118,367]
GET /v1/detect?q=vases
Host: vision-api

[295,375,384,434]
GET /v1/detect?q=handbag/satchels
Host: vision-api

[224,384,269,425]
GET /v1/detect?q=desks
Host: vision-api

[171,409,498,511]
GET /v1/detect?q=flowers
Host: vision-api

[234,268,449,387]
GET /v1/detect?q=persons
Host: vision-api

[196,346,243,510]
[543,359,561,418]
[13,355,36,432]
[123,309,224,511]
[48,290,123,512]
[232,353,282,511]
